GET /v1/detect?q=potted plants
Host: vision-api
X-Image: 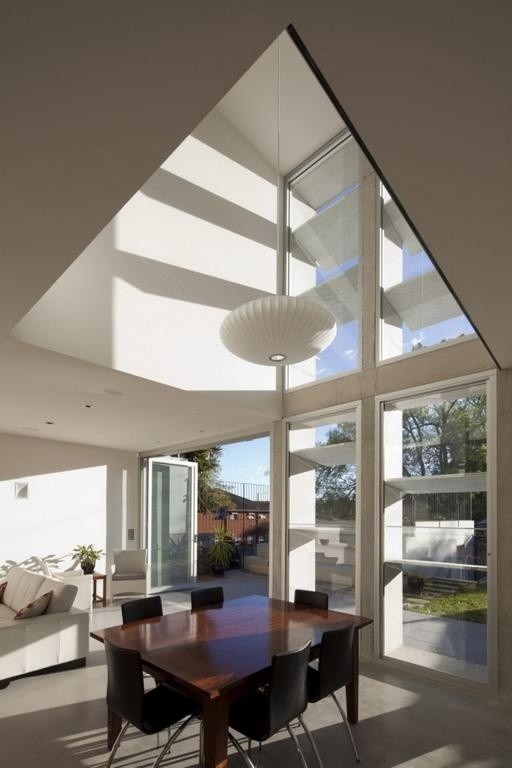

[71,544,103,573]
[207,523,235,576]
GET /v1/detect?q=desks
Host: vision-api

[90,593,374,765]
[92,572,106,607]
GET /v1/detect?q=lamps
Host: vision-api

[219,35,337,365]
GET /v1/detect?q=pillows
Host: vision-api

[15,590,52,619]
[0,581,8,604]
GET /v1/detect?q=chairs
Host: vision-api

[110,549,150,605]
[224,639,314,767]
[190,586,225,609]
[293,588,330,611]
[297,621,362,768]
[121,595,164,625]
[105,638,202,768]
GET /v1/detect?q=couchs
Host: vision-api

[0,567,89,691]
